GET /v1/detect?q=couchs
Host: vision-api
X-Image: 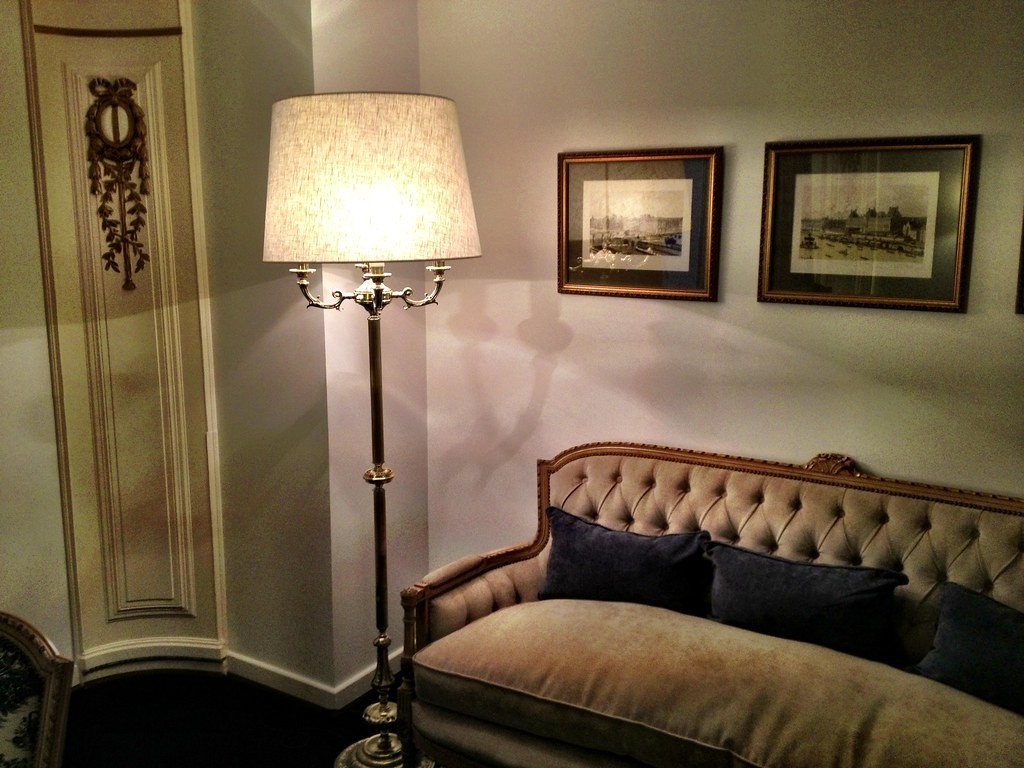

[401,442,1024,767]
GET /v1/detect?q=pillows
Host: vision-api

[534,506,710,619]
[910,583,1024,719]
[705,537,911,665]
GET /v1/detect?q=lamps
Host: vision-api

[262,89,485,316]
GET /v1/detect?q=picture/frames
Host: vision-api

[757,133,983,313]
[556,146,722,302]
